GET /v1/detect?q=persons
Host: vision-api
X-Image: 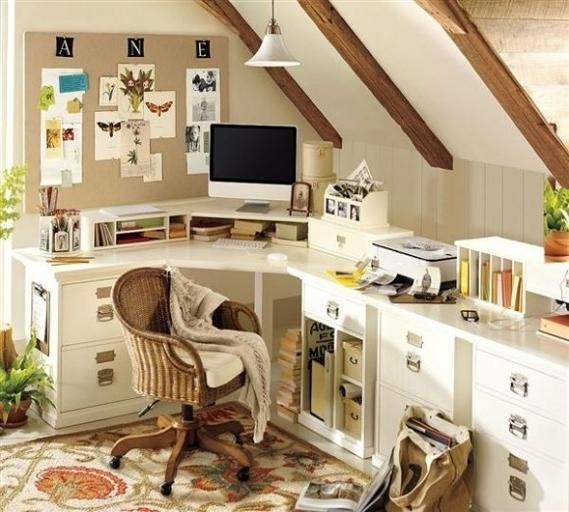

[187,125,200,152]
[199,97,209,120]
[192,71,216,92]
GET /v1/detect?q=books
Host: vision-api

[406,417,456,447]
[117,217,187,245]
[295,445,395,512]
[276,327,301,413]
[95,222,114,247]
[191,220,308,248]
[45,250,96,266]
[538,314,569,341]
[461,261,523,312]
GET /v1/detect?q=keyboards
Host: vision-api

[211,238,268,250]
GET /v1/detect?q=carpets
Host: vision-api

[0,401,373,512]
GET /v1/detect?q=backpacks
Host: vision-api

[383,402,474,512]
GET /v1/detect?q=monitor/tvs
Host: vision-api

[208,122,297,215]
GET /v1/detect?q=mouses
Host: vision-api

[266,252,286,261]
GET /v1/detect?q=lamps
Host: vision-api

[242,0,300,68]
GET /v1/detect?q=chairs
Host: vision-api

[108,266,260,492]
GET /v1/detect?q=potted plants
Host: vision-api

[543,175,569,261]
[0,322,52,428]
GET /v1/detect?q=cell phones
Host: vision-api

[461,310,479,321]
